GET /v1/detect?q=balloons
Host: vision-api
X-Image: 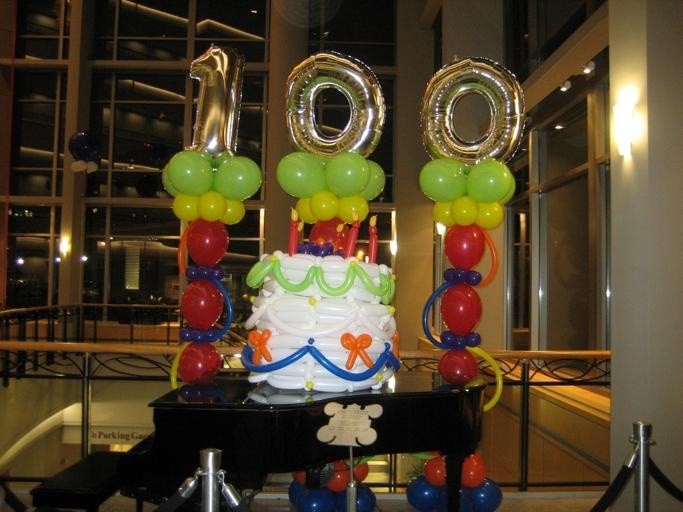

[288,457,376,509]
[240,50,401,381]
[419,57,525,411]
[405,453,502,509]
[69,130,101,169]
[162,42,263,396]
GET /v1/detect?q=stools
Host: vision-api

[29,450,144,512]
